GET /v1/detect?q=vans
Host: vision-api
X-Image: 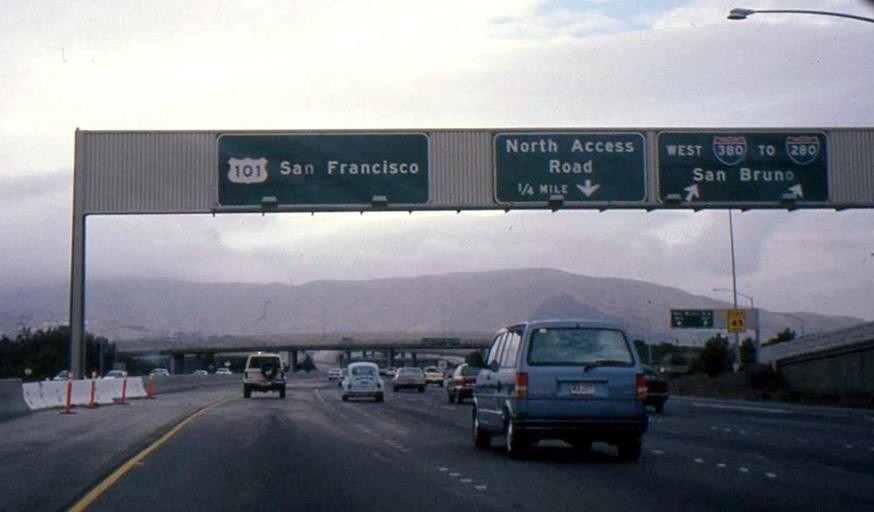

[470,321,646,461]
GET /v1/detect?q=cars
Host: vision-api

[342,363,383,401]
[639,365,669,415]
[424,365,444,387]
[106,370,128,382]
[448,365,480,402]
[328,365,343,380]
[55,369,69,383]
[148,368,169,379]
[394,366,432,393]
[217,368,232,379]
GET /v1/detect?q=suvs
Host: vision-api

[243,353,284,401]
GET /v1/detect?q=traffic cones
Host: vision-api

[147,373,155,399]
[115,378,130,404]
[59,382,76,414]
[87,381,97,407]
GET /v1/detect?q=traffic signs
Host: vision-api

[726,309,746,331]
[670,308,714,328]
[673,309,714,327]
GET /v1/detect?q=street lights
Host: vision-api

[726,8,870,25]
[728,9,874,22]
[713,286,755,311]
